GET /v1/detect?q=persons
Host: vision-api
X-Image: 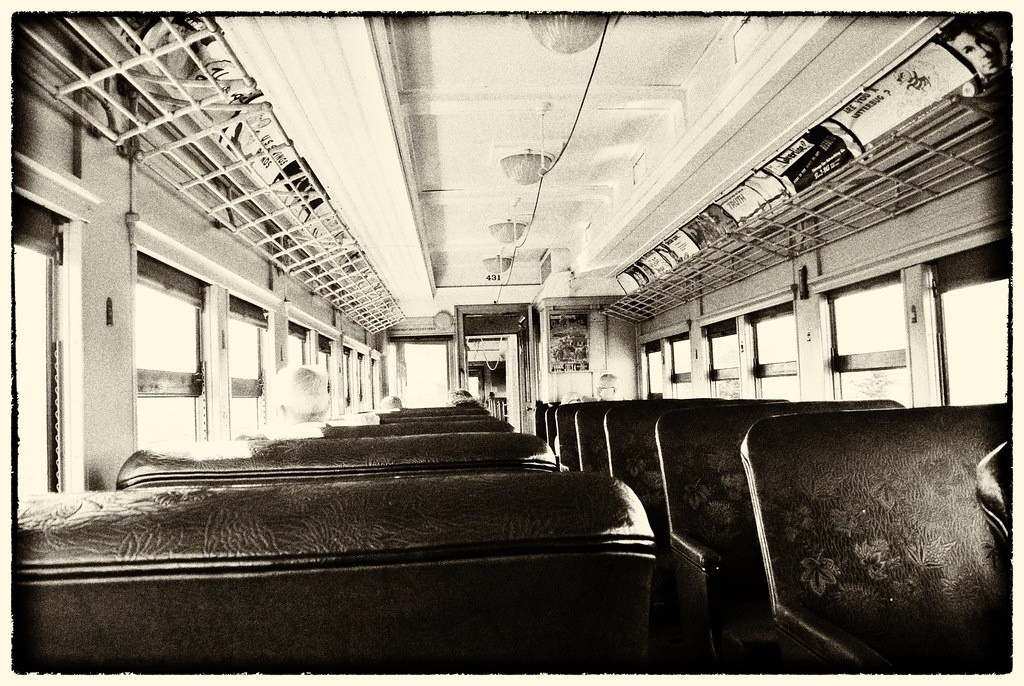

[269,367,379,426]
[488,391,494,398]
[448,390,474,405]
[378,397,402,411]
[946,20,1004,73]
[598,373,616,394]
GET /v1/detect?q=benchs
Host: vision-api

[15,398,1012,674]
